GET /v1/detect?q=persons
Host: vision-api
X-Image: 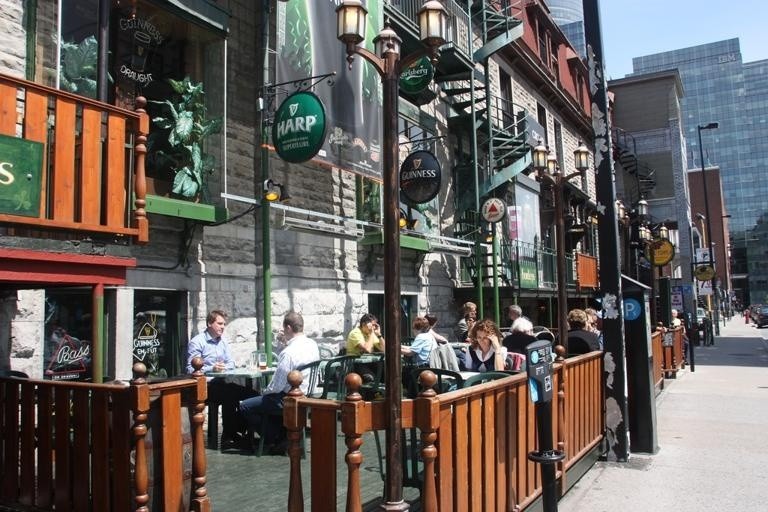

[188,308,262,454]
[235,310,322,456]
[670,307,687,339]
[341,302,603,446]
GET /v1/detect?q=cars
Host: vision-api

[696,307,706,330]
[748,304,767,327]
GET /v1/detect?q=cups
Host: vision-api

[259,353,267,370]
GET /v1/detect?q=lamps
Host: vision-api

[399,208,418,228]
[263,178,292,203]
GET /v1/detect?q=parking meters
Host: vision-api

[525,340,566,512]
[683,310,693,371]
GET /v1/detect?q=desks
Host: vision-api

[205,367,277,449]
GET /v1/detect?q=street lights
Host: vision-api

[532,135,591,358]
[334,0,448,512]
[697,120,719,316]
[613,192,650,292]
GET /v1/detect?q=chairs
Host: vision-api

[254,355,520,482]
